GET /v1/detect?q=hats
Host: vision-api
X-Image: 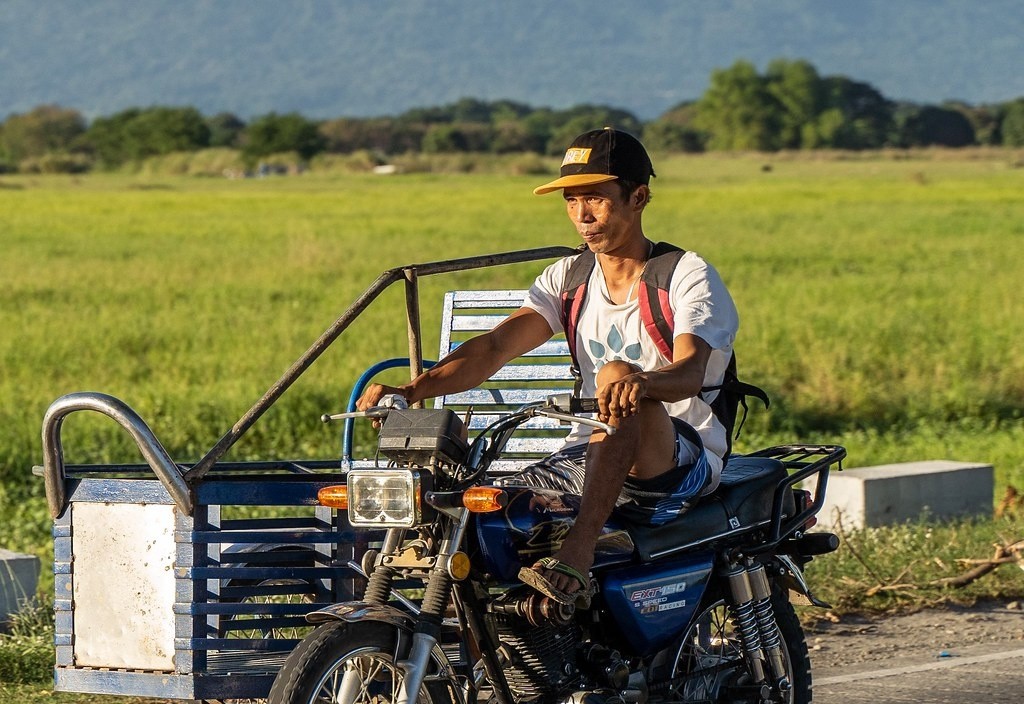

[533,127,655,196]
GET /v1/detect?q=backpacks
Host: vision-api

[559,242,771,461]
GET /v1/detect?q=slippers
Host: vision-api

[519,556,593,610]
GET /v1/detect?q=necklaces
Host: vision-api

[624,243,653,303]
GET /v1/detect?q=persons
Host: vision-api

[356,128,738,604]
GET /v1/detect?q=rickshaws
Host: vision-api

[28,243,849,703]
